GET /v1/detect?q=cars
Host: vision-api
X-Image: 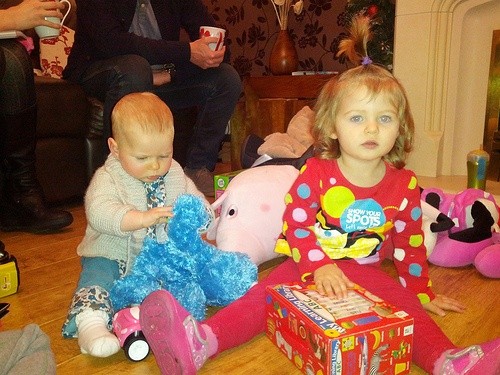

[0,240,21,299]
[111,306,152,363]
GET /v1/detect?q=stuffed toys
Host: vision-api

[109,192,258,323]
[207,165,300,266]
[418,186,500,279]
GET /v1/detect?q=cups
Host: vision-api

[199,25,226,52]
[31,0,71,39]
[0,240,20,297]
[467,150,490,191]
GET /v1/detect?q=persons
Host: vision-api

[140,15,500,375]
[61,92,215,357]
[61,0,242,195]
[0,0,73,234]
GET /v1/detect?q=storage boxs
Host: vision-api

[214,169,251,217]
[264,278,414,375]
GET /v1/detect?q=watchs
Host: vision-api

[163,63,176,82]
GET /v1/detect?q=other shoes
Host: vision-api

[138,289,209,375]
[183,164,215,197]
[438,336,500,375]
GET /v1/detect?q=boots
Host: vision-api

[0,102,74,234]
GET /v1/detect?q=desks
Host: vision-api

[230,73,338,172]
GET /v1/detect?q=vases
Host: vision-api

[269,30,298,75]
